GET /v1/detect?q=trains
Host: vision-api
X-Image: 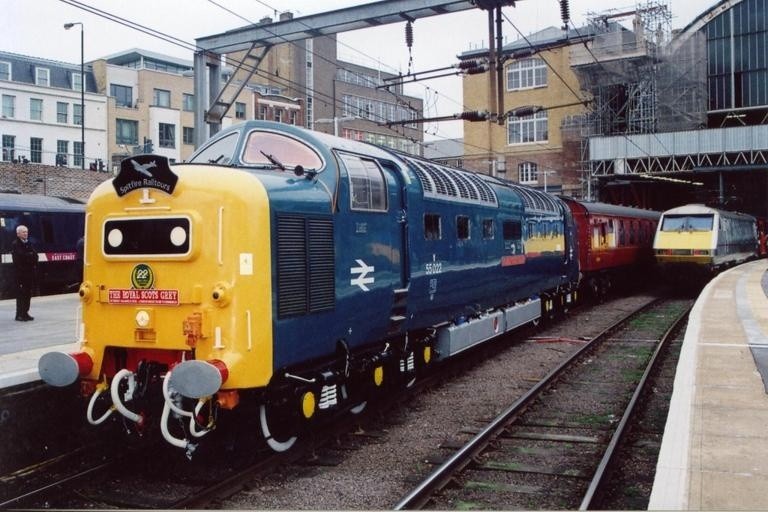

[39,120,661,461]
[651,201,767,284]
[0,188,90,301]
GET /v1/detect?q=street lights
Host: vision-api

[62,20,86,169]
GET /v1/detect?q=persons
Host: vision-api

[11,225,39,321]
